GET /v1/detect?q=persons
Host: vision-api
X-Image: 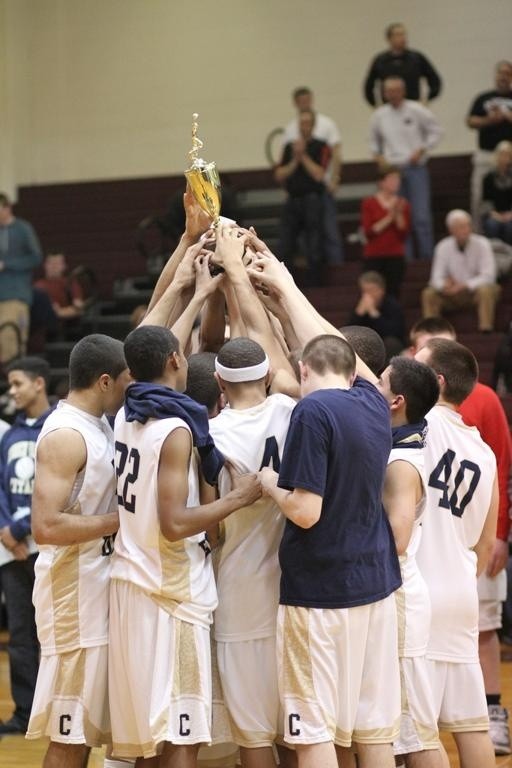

[0,191,43,363]
[279,85,346,267]
[23,210,511,768]
[367,75,443,270]
[273,108,334,286]
[0,357,57,735]
[481,139,512,246]
[468,59,512,229]
[358,168,415,302]
[363,22,442,106]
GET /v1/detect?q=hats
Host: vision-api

[0,179,19,205]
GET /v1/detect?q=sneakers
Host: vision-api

[1,712,29,732]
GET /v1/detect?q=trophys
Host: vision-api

[182,110,253,276]
